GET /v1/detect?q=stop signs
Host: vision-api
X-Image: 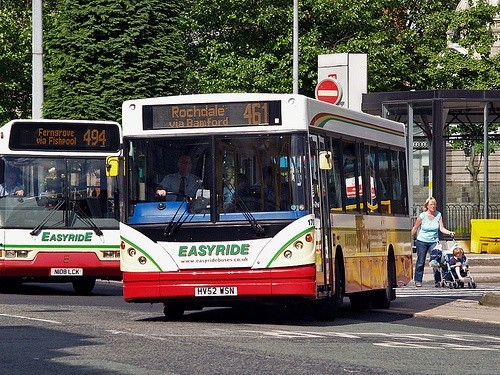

[314,77,343,104]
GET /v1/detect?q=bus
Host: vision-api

[105,92,414,322]
[0,119,132,295]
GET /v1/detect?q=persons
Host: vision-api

[224,172,253,210]
[71,188,113,211]
[449,247,473,285]
[156,153,204,205]
[223,157,402,213]
[0,172,25,197]
[408,197,455,287]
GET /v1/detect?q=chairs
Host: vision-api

[245,183,290,211]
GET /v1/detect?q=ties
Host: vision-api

[176,176,186,201]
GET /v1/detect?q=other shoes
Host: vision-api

[416,283,422,287]
[435,282,440,287]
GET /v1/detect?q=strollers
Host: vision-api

[429,233,477,289]
